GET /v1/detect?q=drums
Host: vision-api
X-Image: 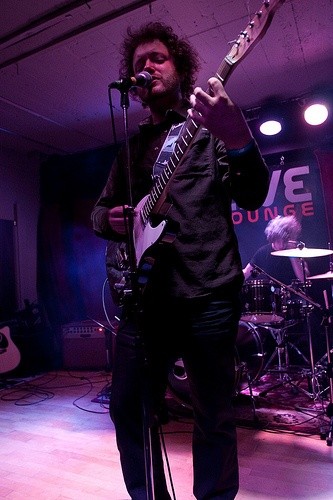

[239,279,286,323]
[284,285,308,323]
[166,322,265,408]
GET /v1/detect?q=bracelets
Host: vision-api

[226,138,254,154]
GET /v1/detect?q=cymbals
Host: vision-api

[305,271,333,280]
[270,249,333,258]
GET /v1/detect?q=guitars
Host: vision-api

[104,0,286,310]
[0,326,22,373]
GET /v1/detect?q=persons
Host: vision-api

[90,22,270,500]
[242,214,312,286]
